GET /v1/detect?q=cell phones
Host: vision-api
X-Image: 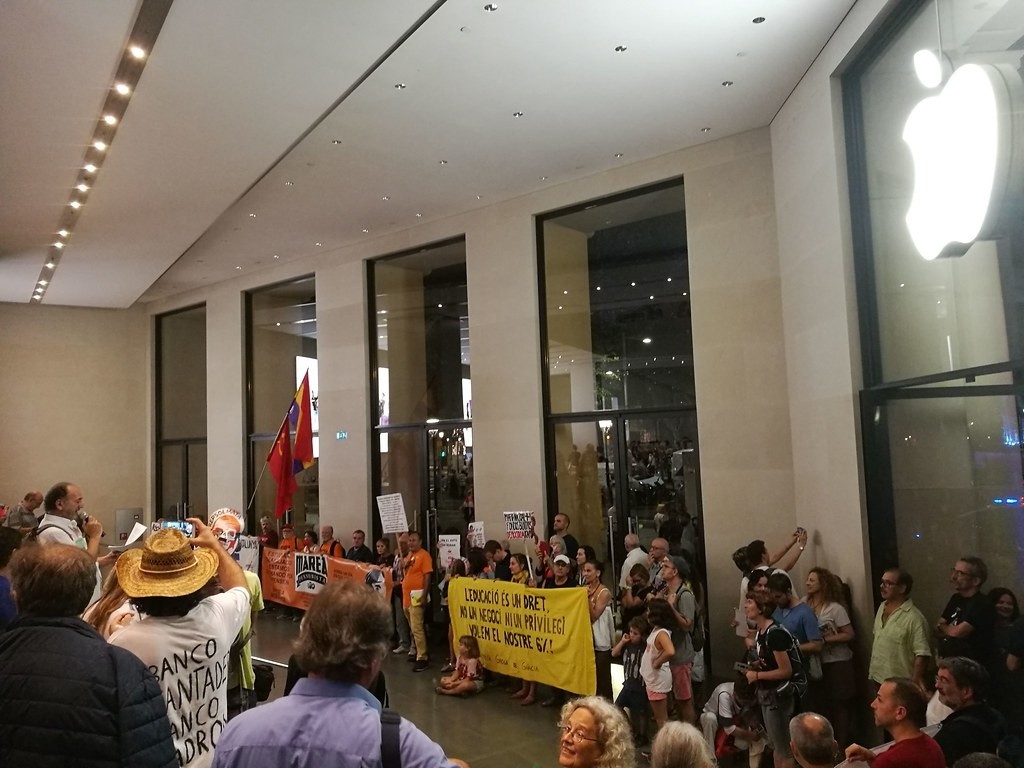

[160,521,196,538]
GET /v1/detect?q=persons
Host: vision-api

[433,428,709,605]
[0,480,1024,768]
[467,525,476,547]
[282,504,309,526]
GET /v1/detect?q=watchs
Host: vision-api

[797,543,804,551]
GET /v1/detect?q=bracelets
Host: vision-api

[756,672,758,680]
[625,586,632,590]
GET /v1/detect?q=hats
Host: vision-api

[281,524,294,529]
[554,555,570,564]
[666,554,690,580]
[116,527,220,598]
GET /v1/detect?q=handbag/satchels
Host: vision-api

[716,691,749,759]
[252,664,275,702]
[808,654,823,682]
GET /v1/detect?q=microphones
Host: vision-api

[79,512,105,538]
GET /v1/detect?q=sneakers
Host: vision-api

[392,646,417,656]
[408,656,429,671]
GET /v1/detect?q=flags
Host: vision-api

[269,372,314,519]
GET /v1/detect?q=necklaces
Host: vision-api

[812,599,822,609]
[591,583,600,595]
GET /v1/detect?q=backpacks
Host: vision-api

[755,623,808,703]
[320,541,346,558]
[952,707,1011,755]
[674,588,706,652]
[22,524,73,545]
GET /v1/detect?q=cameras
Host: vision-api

[797,527,803,533]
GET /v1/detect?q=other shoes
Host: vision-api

[635,733,649,748]
[440,665,455,672]
[435,687,443,695]
[489,678,557,706]
[277,614,301,621]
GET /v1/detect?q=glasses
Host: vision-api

[881,581,900,588]
[651,545,665,550]
[951,568,975,578]
[633,578,642,583]
[935,675,958,686]
[562,725,599,743]
[662,564,676,569]
